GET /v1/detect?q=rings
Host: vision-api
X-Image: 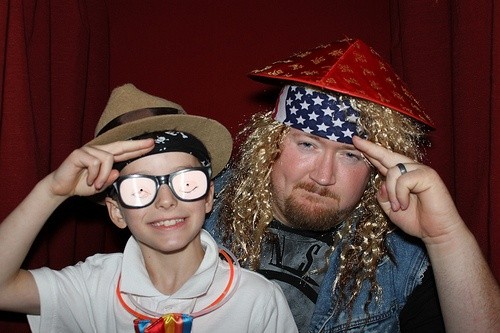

[396,163,407,174]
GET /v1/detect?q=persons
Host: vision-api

[0,84,299,333]
[202,36,500,333]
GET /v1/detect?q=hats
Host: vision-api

[85,83,234,178]
[249,33,438,127]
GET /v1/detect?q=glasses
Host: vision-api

[113,163,212,209]
